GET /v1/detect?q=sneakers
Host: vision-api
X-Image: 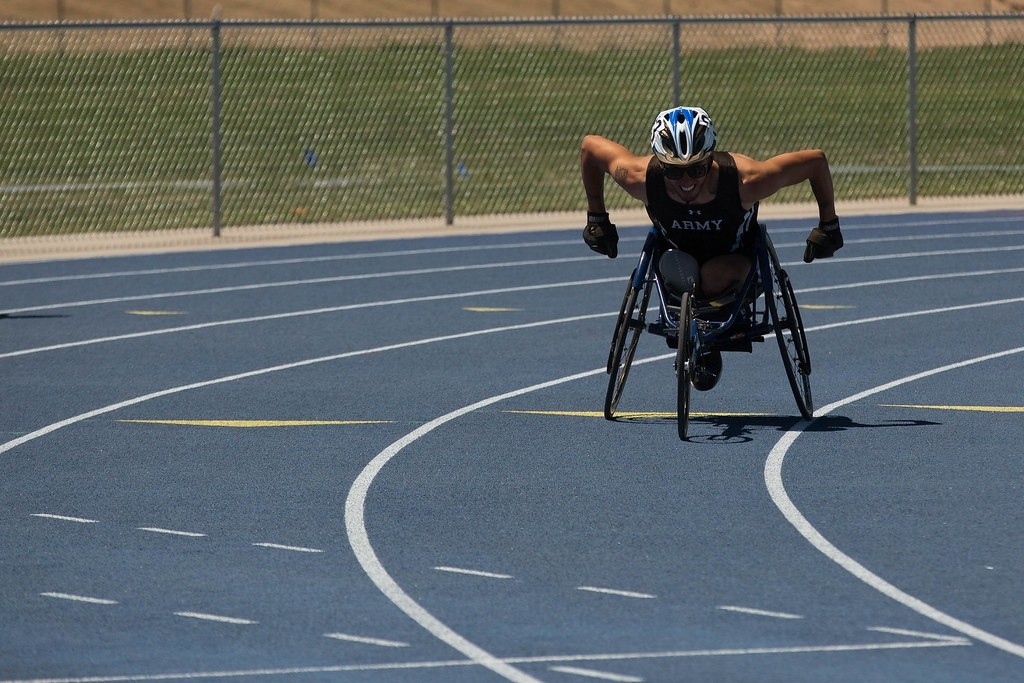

[688,332,722,391]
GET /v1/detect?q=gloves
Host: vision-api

[803,217,844,263]
[583,210,618,259]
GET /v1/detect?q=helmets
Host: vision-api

[652,106,717,165]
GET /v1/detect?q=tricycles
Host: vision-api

[603,223,813,441]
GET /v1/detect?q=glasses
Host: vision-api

[659,153,713,179]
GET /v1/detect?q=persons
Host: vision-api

[582,106,843,315]
